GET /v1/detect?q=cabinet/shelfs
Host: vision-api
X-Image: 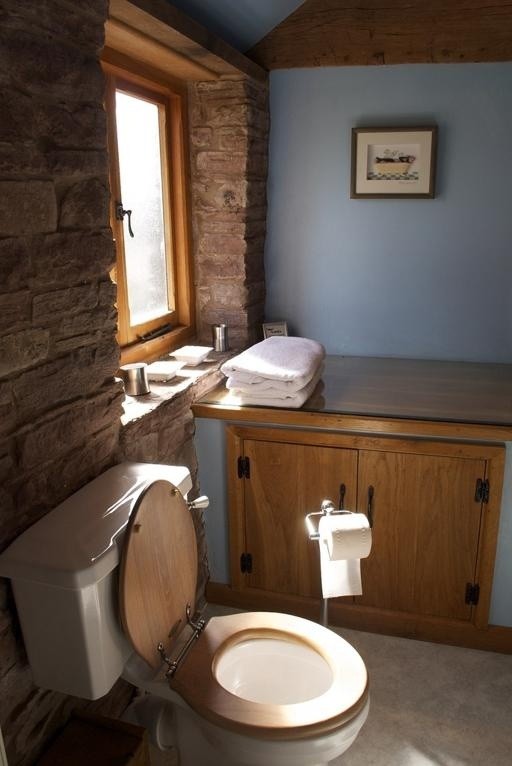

[190,354,512,656]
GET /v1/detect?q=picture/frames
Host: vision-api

[262,321,289,340]
[349,125,438,200]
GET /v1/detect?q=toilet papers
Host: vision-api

[319,514,372,599]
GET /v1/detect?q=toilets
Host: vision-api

[1,461,370,766]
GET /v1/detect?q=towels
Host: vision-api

[220,335,326,410]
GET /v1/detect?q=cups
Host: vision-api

[212,323,228,353]
[119,363,150,396]
